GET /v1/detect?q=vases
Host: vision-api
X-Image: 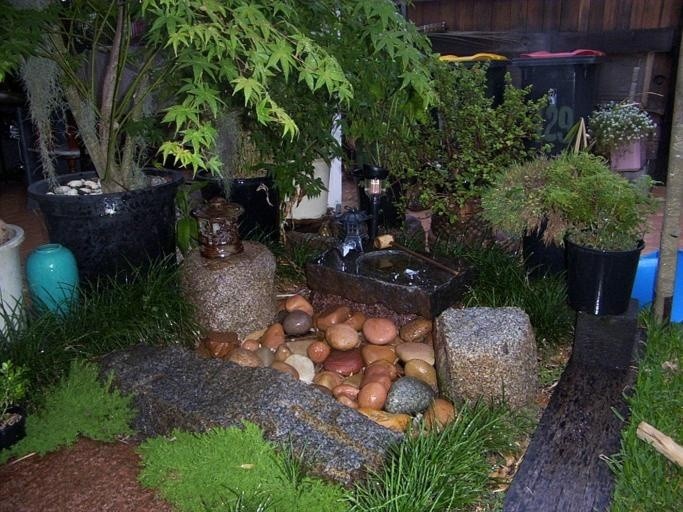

[1,223,26,334]
[607,141,648,172]
[28,243,79,316]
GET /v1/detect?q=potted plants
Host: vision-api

[532,150,665,315]
[1,1,442,305]
[383,58,556,253]
[481,156,567,276]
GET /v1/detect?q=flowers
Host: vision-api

[585,101,658,142]
